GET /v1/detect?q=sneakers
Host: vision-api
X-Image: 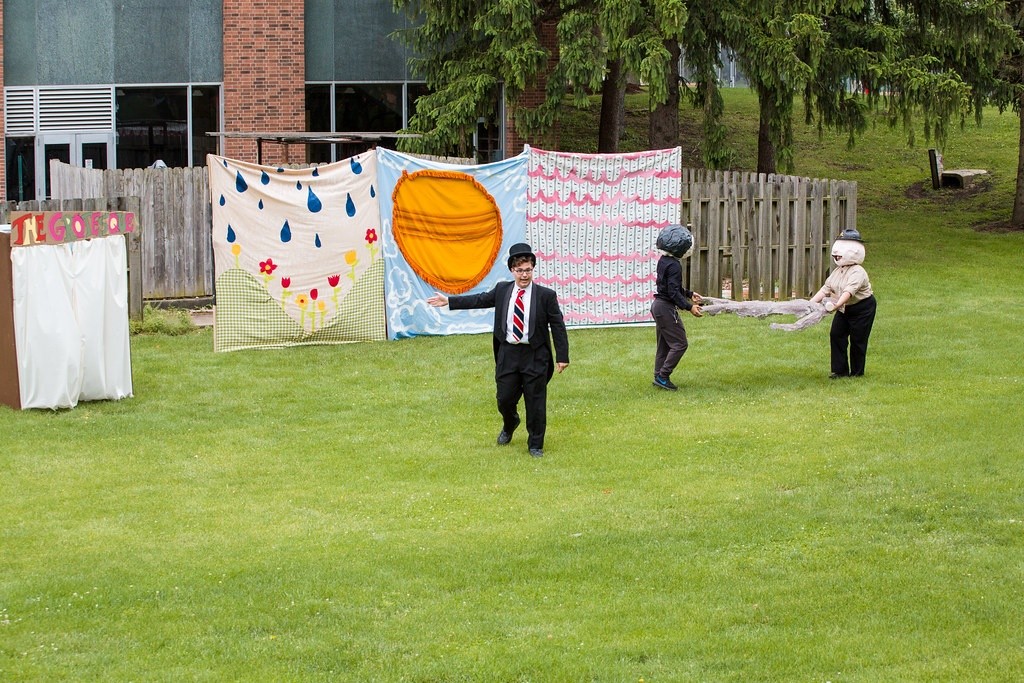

[652,375,677,391]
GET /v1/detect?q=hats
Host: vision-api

[834,229,866,243]
[508,243,536,270]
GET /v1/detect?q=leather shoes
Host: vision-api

[497,418,521,445]
[529,449,543,458]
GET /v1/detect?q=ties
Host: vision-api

[511,289,525,342]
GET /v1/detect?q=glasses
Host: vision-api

[513,267,534,275]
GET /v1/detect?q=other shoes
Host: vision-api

[828,373,864,379]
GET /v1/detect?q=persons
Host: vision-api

[809,229,876,378]
[427,243,569,456]
[650,224,703,390]
